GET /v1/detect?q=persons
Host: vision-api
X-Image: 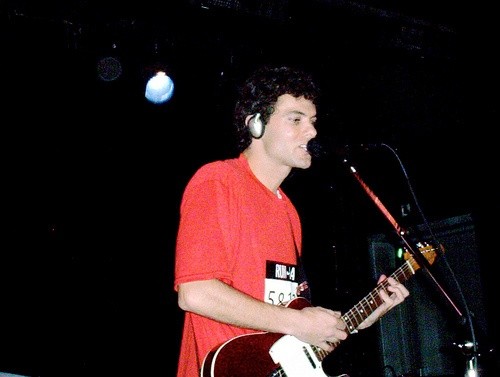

[175,66,410,377]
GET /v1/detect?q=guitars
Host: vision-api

[201,242,445,377]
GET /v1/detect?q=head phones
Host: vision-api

[248,110,265,138]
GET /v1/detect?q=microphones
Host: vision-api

[307,138,383,158]
